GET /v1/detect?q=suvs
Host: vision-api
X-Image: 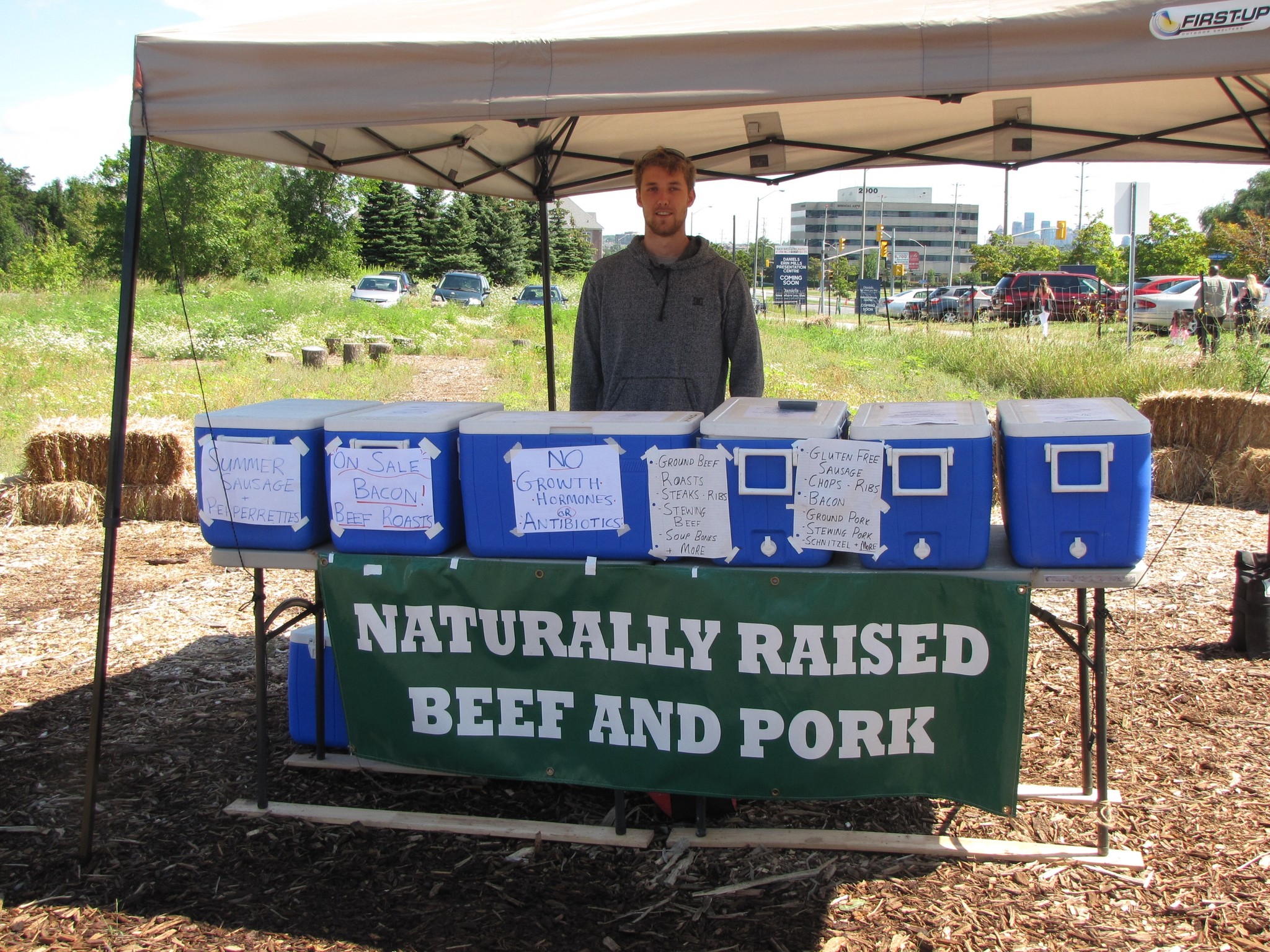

[991,270,1121,327]
[431,270,493,308]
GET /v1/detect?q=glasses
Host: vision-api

[642,148,686,161]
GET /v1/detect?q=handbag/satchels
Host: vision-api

[1034,288,1042,315]
[1045,291,1056,311]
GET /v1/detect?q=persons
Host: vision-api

[1229,274,1263,349]
[1194,265,1232,358]
[568,147,763,418]
[1161,309,1195,354]
[1032,277,1055,342]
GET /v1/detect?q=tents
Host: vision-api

[76,0,1270,859]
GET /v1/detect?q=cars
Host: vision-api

[512,284,569,307]
[874,285,997,324]
[350,271,419,308]
[1111,274,1270,337]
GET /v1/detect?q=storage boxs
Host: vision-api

[699,396,851,567]
[194,398,384,551]
[324,401,504,557]
[849,400,994,571]
[996,396,1153,569]
[457,411,704,560]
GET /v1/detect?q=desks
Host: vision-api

[210,525,1148,871]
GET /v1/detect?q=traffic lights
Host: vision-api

[881,241,887,257]
[877,223,884,242]
[839,237,846,250]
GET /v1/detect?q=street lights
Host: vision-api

[1073,162,1091,236]
[908,237,927,288]
[690,205,713,236]
[946,182,965,286]
[752,189,785,299]
